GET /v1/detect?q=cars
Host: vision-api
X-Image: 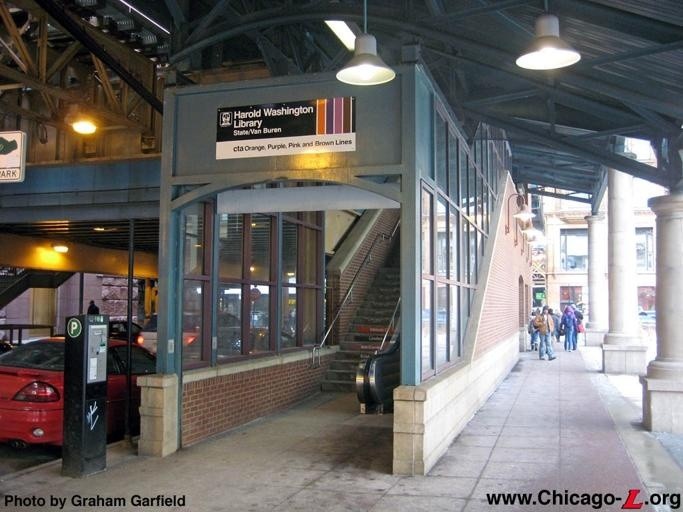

[136,309,240,363]
[108,320,142,343]
[251,327,294,350]
[0,333,156,448]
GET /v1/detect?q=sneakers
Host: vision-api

[539,357,556,360]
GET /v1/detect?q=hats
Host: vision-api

[543,305,548,309]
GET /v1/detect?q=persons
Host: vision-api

[86,300,99,315]
[527,303,583,352]
[533,305,558,361]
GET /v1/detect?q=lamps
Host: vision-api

[336,0,395,85]
[515,218,545,266]
[515,0,581,71]
[53,243,69,253]
[64,103,97,134]
[505,193,536,236]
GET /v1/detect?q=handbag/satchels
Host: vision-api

[559,329,565,336]
[576,324,585,333]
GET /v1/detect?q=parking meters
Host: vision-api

[60,313,109,479]
